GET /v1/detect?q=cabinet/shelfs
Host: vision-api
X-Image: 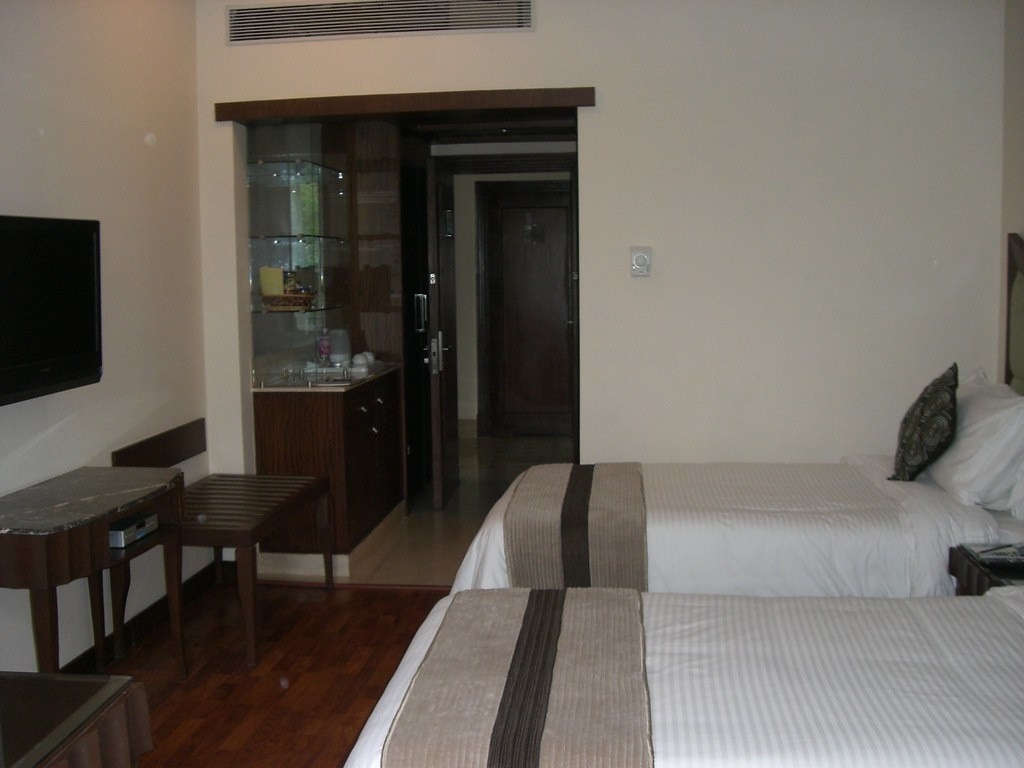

[251,364,405,555]
[247,157,356,365]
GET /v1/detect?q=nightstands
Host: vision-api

[948,543,1024,598]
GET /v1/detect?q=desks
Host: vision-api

[113,473,335,667]
[0,467,189,677]
[0,672,154,768]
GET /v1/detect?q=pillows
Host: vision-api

[981,381,1024,511]
[886,362,959,481]
[927,351,1024,506]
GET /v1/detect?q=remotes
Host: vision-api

[979,552,1024,564]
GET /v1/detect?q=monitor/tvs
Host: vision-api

[0,215,103,406]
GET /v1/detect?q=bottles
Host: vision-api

[318,327,330,361]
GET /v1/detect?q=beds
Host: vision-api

[448,234,1024,597]
[341,583,1024,768]
